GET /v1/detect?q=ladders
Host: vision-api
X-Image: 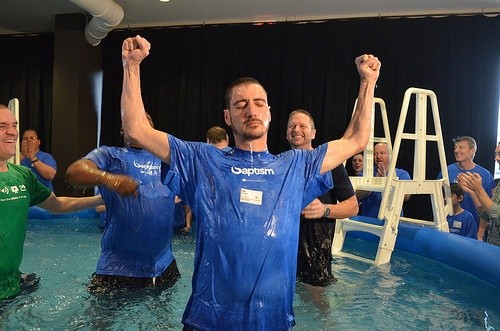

[6,98,20,166]
[331,87,453,264]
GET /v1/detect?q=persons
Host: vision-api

[20,129,57,193]
[442,183,477,240]
[435,136,496,242]
[457,141,500,246]
[0,103,104,299]
[207,126,234,155]
[64,111,181,285]
[360,142,411,219]
[121,35,381,331]
[94,186,109,220]
[350,151,375,200]
[286,110,359,287]
[175,195,192,233]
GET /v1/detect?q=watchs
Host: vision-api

[31,157,38,164]
[324,205,330,218]
